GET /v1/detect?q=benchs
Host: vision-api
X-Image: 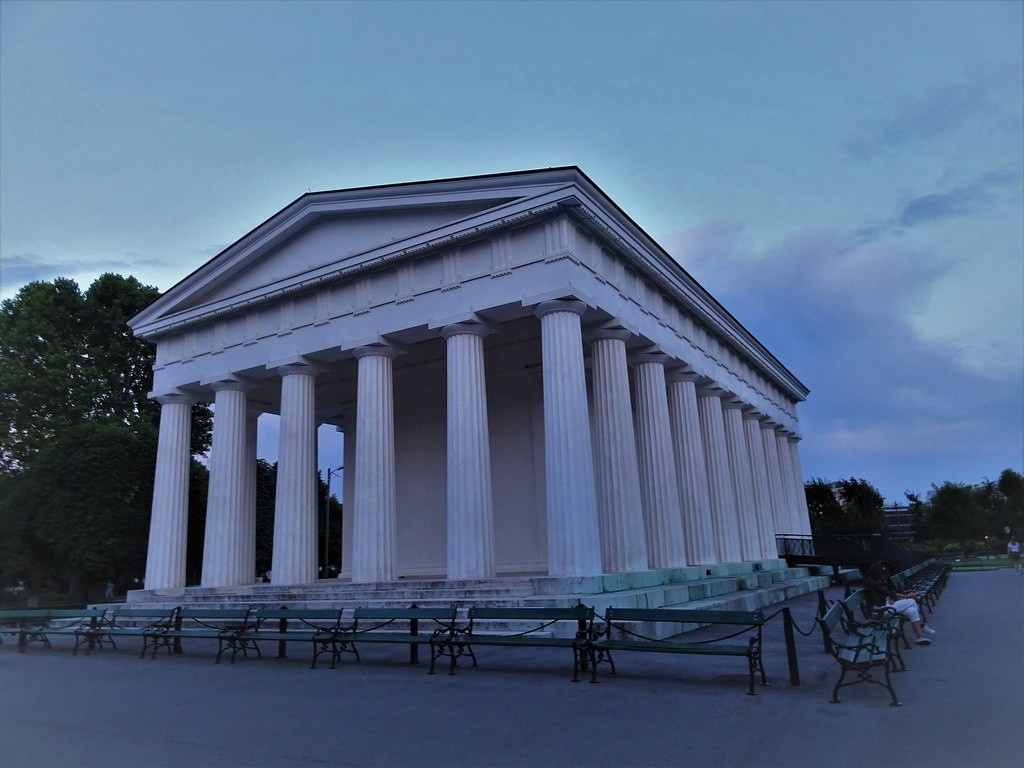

[0,558,955,710]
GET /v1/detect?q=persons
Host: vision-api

[1007,536,1021,568]
[864,560,936,644]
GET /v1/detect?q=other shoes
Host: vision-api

[1015,568,1023,576]
[921,624,936,634]
[914,637,931,645]
